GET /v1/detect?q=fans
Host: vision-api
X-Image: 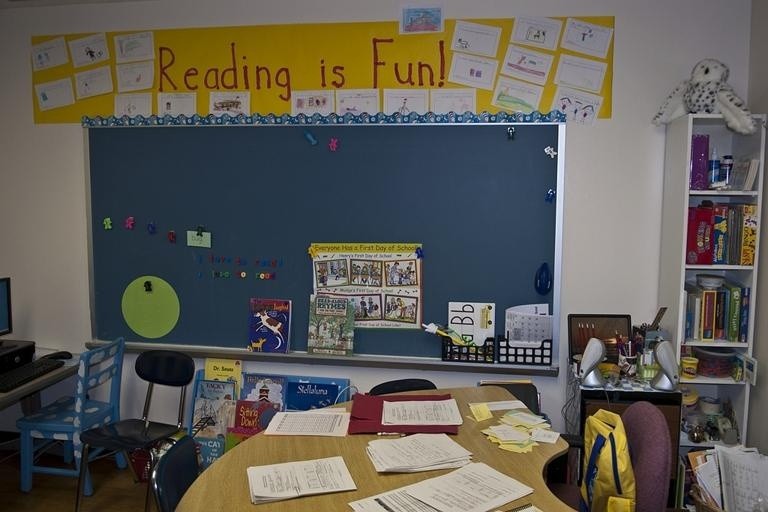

[81,115,565,377]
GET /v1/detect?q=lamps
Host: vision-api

[16,337,127,496]
[621,401,672,512]
[75,350,195,511]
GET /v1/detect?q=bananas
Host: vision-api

[0,358,65,393]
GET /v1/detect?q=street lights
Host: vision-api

[579,409,637,512]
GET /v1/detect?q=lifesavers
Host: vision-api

[0,340,35,374]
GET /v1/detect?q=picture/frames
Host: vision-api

[1,346,81,417]
[176,384,578,512]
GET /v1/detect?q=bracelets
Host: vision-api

[695,274,724,290]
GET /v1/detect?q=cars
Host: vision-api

[617,352,638,377]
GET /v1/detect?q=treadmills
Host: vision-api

[651,307,667,330]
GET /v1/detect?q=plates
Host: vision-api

[658,112,768,511]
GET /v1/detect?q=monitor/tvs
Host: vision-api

[191,357,280,474]
[728,157,760,192]
[307,294,354,357]
[247,298,292,353]
[684,290,701,340]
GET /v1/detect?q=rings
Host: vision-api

[419,323,483,355]
[614,329,629,357]
[376,432,413,438]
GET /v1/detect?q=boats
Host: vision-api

[578,337,604,388]
[651,341,680,391]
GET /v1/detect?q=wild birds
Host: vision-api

[568,314,633,375]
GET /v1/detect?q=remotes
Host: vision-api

[48,351,72,360]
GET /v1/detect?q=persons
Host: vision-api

[318,262,415,320]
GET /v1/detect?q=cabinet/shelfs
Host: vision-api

[708,148,733,183]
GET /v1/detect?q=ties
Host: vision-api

[0,277,17,351]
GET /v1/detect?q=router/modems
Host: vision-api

[699,396,722,414]
